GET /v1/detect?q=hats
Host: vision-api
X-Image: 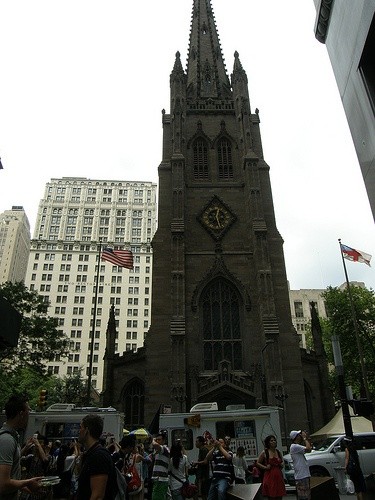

[289,430,302,440]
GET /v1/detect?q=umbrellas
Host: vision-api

[129,427,151,439]
[123,429,130,436]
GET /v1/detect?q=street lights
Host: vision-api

[261,339,276,406]
[330,335,353,438]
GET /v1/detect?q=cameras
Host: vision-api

[137,445,141,450]
[33,434,38,440]
[212,440,214,443]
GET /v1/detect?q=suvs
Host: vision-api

[283,430,375,494]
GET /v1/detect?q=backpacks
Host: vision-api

[123,455,142,495]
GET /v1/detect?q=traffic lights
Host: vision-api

[40,389,48,407]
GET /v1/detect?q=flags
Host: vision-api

[101,248,134,270]
[341,244,372,266]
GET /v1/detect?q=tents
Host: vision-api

[308,403,374,438]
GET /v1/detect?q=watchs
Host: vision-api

[303,438,306,440]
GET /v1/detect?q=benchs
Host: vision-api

[225,483,263,500]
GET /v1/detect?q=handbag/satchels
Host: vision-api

[345,474,355,493]
[181,482,198,498]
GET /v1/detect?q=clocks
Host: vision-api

[204,206,231,230]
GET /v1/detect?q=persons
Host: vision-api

[45,437,87,500]
[343,436,366,500]
[21,430,51,496]
[289,430,312,500]
[256,435,287,500]
[106,436,153,500]
[151,435,171,500]
[79,415,117,500]
[231,447,248,484]
[0,394,46,500]
[169,443,192,500]
[203,431,233,500]
[248,460,259,483]
[194,436,209,500]
